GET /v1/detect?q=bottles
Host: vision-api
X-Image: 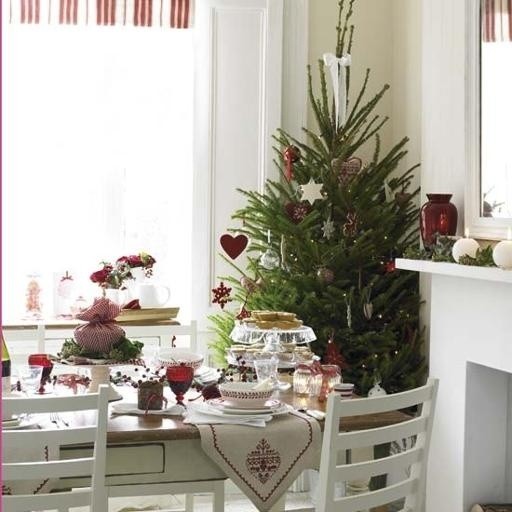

[138,380,163,411]
[418,190,457,254]
[1,326,13,399]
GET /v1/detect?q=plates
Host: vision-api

[221,404,273,415]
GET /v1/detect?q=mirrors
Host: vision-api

[462,0,512,241]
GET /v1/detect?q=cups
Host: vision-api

[16,364,43,396]
[252,348,354,405]
[133,281,172,309]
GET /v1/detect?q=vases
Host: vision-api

[420,194,457,259]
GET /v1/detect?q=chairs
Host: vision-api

[285,376,440,512]
[38,321,225,511]
[2,383,110,512]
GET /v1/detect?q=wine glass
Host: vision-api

[167,366,195,409]
[27,353,54,395]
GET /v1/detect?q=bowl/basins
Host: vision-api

[157,350,204,375]
[218,382,273,408]
